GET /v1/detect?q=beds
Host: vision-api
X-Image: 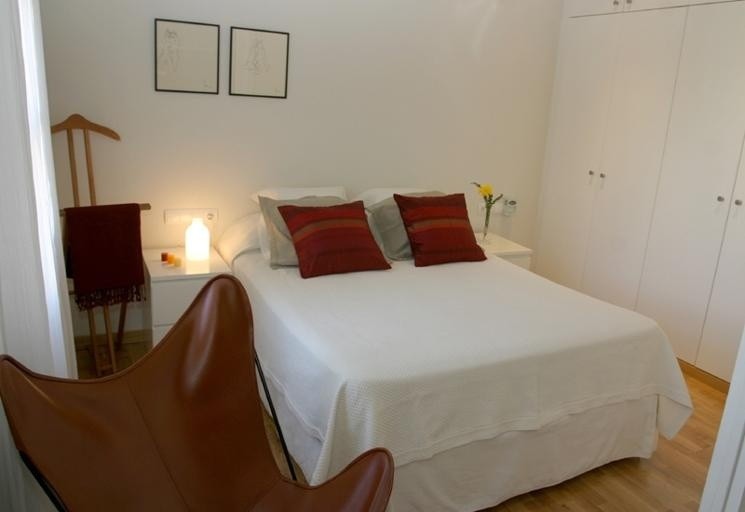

[225,212,667,512]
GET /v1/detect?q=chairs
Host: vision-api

[0,274,394,511]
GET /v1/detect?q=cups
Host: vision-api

[159,251,182,266]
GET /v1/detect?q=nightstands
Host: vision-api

[137,243,234,351]
[470,231,534,272]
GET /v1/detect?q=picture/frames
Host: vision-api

[154,17,290,100]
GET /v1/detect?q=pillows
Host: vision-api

[256,190,487,279]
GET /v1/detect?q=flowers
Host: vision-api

[471,179,504,236]
